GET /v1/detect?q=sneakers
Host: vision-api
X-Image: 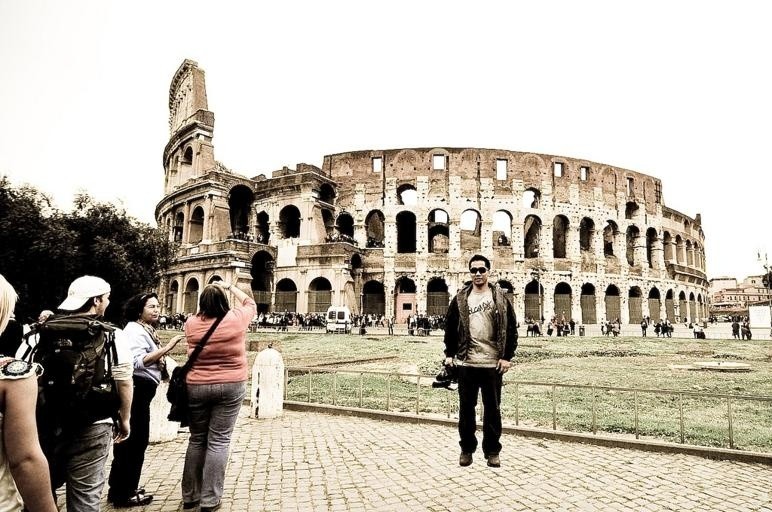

[182,500,199,511]
[484,451,500,468]
[459,446,476,467]
[203,500,221,512]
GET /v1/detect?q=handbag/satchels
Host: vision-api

[165,366,188,404]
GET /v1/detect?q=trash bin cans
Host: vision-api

[249,348,285,419]
[579,326,585,336]
[147,356,180,445]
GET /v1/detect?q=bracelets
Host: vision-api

[226,282,236,289]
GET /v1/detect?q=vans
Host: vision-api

[325,305,353,334]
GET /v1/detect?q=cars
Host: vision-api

[716,316,729,322]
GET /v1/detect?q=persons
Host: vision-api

[324,230,361,248]
[523,313,576,338]
[354,309,447,335]
[441,253,520,471]
[223,228,265,245]
[257,308,327,333]
[181,278,256,510]
[637,313,675,338]
[599,319,623,337]
[683,316,706,339]
[710,313,751,340]
[1,273,184,512]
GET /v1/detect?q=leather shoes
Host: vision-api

[114,493,151,508]
[133,489,145,496]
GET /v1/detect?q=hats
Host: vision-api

[57,273,112,311]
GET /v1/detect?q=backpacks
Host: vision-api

[31,313,112,395]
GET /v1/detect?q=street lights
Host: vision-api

[533,242,542,330]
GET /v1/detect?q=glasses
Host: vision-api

[468,267,488,273]
